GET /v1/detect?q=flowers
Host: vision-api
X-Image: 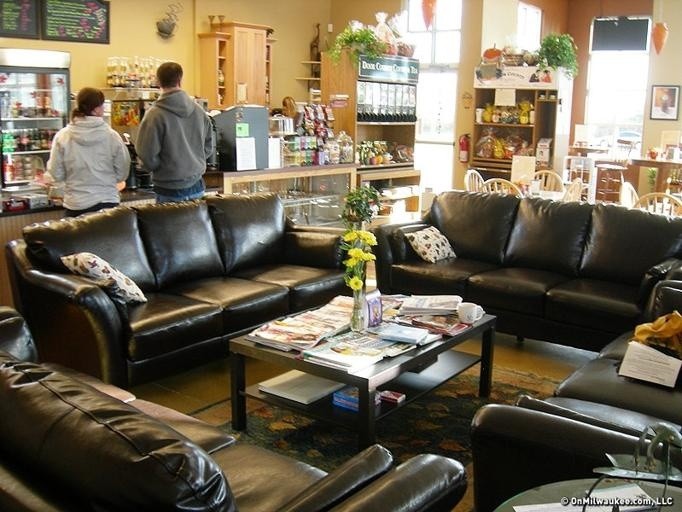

[338,230,378,329]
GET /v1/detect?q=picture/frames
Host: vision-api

[649,84,680,120]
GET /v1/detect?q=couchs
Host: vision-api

[4,216,367,389]
[469,279,682,512]
[367,205,682,353]
[0,305,468,512]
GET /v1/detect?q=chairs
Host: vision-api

[463,168,682,217]
[595,138,635,206]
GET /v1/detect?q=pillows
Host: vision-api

[0,349,395,512]
[634,257,682,306]
[579,200,682,289]
[403,225,458,265]
[430,190,521,269]
[22,191,288,322]
[504,194,595,278]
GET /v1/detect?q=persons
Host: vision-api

[541,70,553,83]
[282,96,304,135]
[45,86,131,218]
[135,60,215,205]
[529,70,541,83]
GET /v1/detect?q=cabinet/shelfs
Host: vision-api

[197,19,423,229]
[468,66,575,174]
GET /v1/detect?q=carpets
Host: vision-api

[217,357,567,512]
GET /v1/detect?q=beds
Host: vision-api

[493,477,682,512]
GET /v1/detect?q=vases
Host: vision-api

[350,288,370,335]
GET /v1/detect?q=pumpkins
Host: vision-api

[483,43,502,59]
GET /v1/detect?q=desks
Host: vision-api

[227,294,498,453]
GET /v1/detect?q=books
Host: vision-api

[242,292,475,411]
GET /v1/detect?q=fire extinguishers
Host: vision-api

[458,133,471,163]
